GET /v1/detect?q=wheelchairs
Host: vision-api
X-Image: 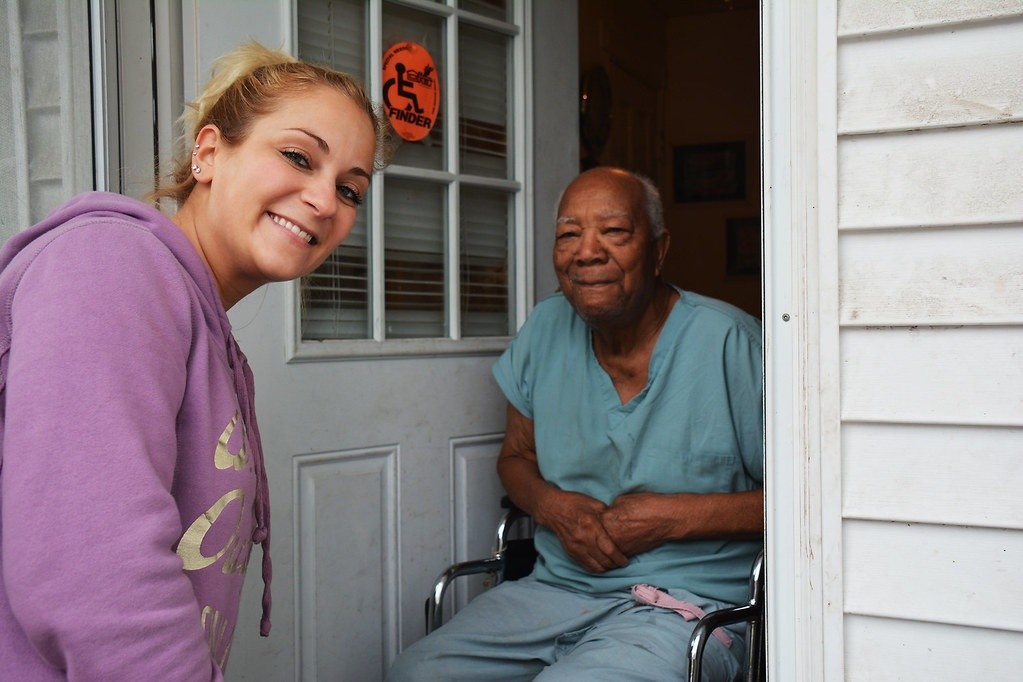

[422,492,767,681]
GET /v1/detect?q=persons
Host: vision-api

[0,33,395,682]
[385,165,760,681]
